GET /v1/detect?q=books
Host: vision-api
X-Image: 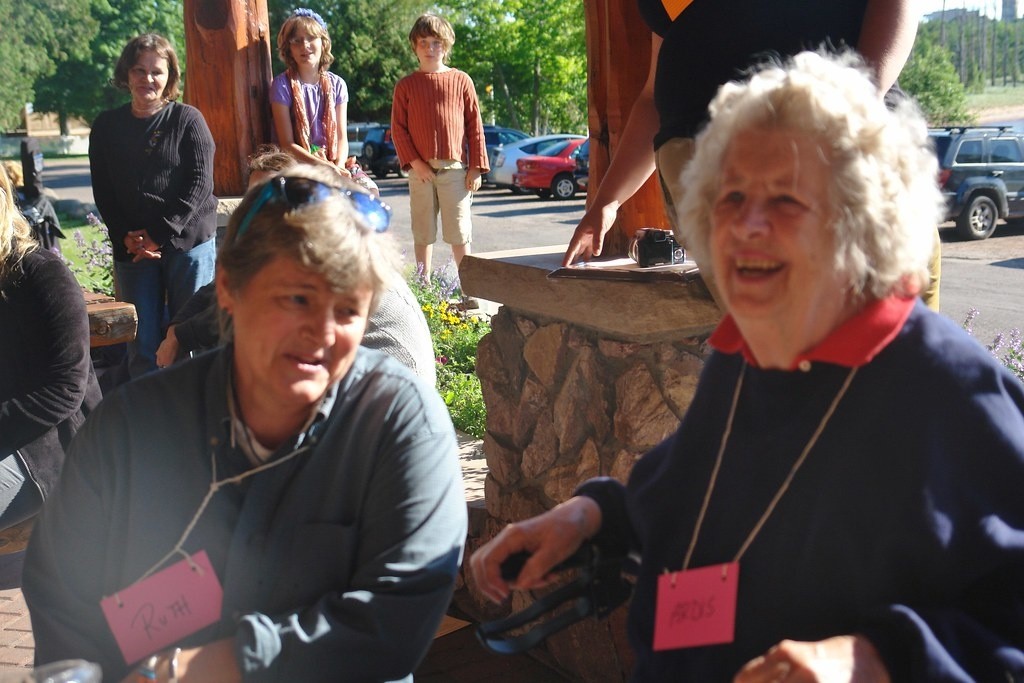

[544,254,702,285]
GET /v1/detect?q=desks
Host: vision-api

[80,284,138,347]
[458,244,726,545]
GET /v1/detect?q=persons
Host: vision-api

[20,172,467,683]
[558,0,931,267]
[2,159,63,256]
[88,34,219,385]
[0,160,109,532]
[390,14,492,310]
[467,49,1023,683]
[151,148,438,387]
[269,7,384,203]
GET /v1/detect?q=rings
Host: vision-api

[506,523,513,528]
[162,365,165,368]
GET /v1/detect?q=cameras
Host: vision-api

[634,228,686,266]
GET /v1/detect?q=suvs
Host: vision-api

[361,123,411,180]
[347,122,383,171]
[925,126,1023,241]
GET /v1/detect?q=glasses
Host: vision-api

[232,176,394,248]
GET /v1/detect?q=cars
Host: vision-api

[572,136,592,190]
[511,137,592,203]
[465,122,532,185]
[488,135,588,195]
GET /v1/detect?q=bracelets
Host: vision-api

[144,643,181,683]
